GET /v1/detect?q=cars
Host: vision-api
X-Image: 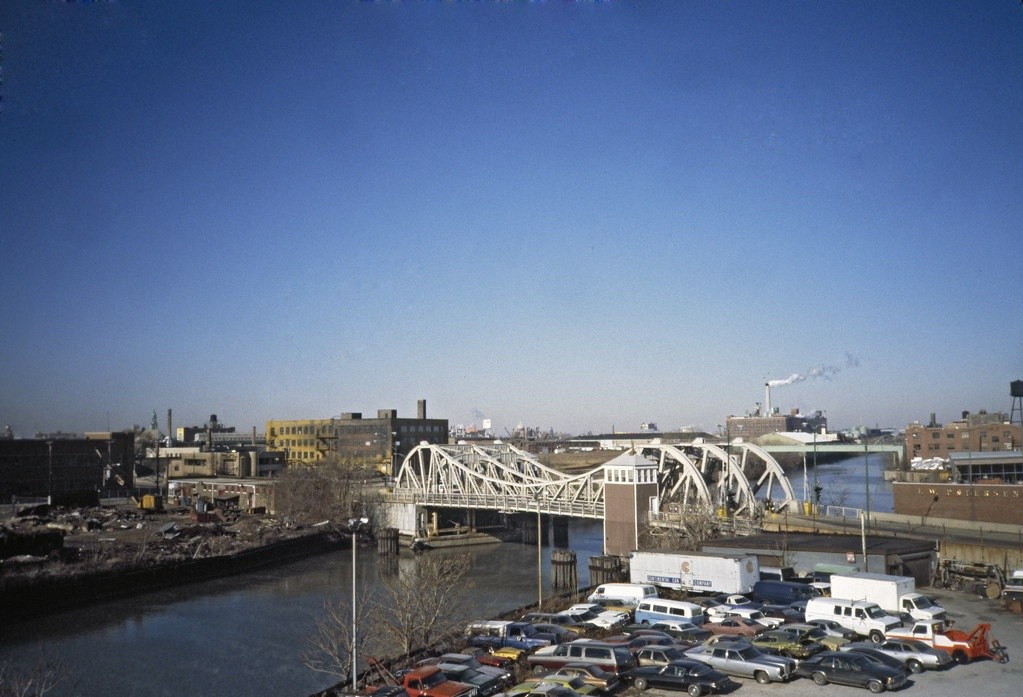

[632,621,730,697]
[367,603,640,697]
[686,594,953,693]
[1001,570,1023,598]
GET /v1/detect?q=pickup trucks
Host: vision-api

[885,620,1008,665]
[364,664,479,697]
[462,621,562,649]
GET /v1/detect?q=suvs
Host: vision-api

[527,639,638,680]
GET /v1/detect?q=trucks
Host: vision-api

[588,584,659,605]
[84,432,112,440]
[628,550,759,595]
[830,572,946,622]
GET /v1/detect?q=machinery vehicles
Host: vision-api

[95,449,167,514]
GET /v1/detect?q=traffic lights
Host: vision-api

[817,487,822,501]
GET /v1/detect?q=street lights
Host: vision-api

[534,491,550,612]
[47,441,54,506]
[347,518,369,693]
[802,422,825,503]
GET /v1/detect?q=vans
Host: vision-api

[635,598,704,628]
[753,567,830,607]
[805,597,903,643]
